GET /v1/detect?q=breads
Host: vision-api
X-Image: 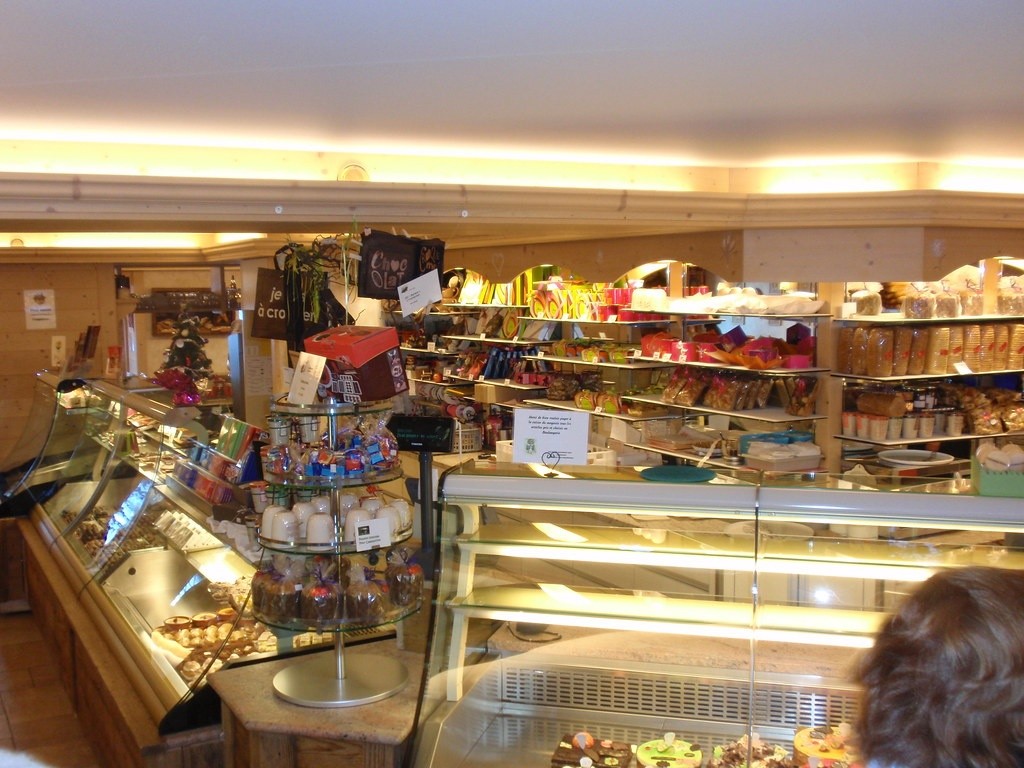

[840,290,1024,377]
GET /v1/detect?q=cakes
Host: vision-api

[551,724,869,768]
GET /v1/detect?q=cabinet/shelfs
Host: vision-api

[390,301,1023,605]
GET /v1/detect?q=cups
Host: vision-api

[841,411,964,441]
[261,504,301,549]
[260,445,274,482]
[306,512,335,552]
[249,481,337,514]
[345,507,372,542]
[291,501,318,539]
[721,439,745,466]
[361,497,412,538]
[266,415,293,446]
[298,414,321,443]
[310,493,360,529]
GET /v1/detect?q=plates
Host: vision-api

[740,430,813,454]
[691,444,722,456]
[640,465,717,483]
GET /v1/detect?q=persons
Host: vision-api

[848,566,1024,768]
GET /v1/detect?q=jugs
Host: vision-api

[433,286,460,313]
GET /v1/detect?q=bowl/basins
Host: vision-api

[838,323,1024,377]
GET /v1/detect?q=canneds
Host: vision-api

[902,380,936,408]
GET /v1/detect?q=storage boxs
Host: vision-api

[495,440,616,466]
[304,325,409,407]
[454,428,483,453]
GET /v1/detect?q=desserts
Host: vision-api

[253,562,424,626]
[283,425,398,478]
